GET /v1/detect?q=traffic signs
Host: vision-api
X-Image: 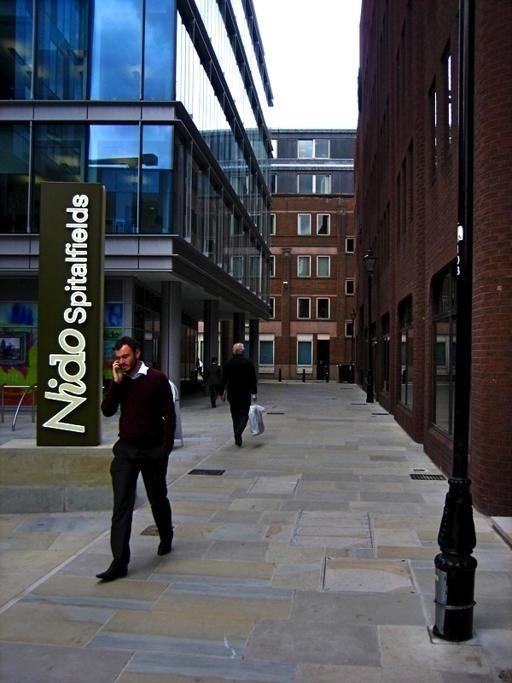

[350,308,357,383]
[363,247,378,403]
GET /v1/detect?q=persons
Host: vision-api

[204,357,221,407]
[94,333,176,582]
[219,342,257,445]
[197,358,204,378]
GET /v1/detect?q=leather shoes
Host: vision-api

[96,562,127,580]
[158,537,172,554]
